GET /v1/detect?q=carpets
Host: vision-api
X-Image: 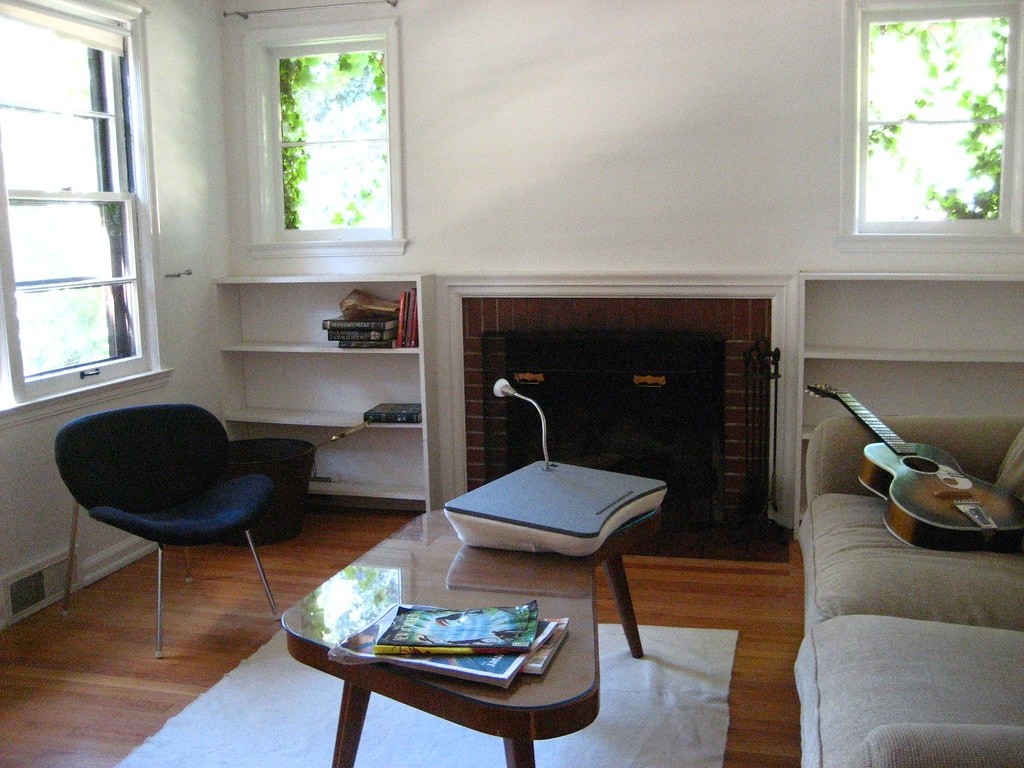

[115,617,738,768]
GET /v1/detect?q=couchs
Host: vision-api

[790,410,1024,768]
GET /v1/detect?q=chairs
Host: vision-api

[54,404,279,659]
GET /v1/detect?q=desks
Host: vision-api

[281,508,643,767]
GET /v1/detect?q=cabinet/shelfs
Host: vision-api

[216,273,441,514]
[794,271,1024,541]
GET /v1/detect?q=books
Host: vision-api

[328,599,569,689]
[322,287,418,348]
[364,403,421,423]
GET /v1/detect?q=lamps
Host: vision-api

[494,378,555,471]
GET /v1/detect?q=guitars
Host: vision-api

[802,379,1024,555]
